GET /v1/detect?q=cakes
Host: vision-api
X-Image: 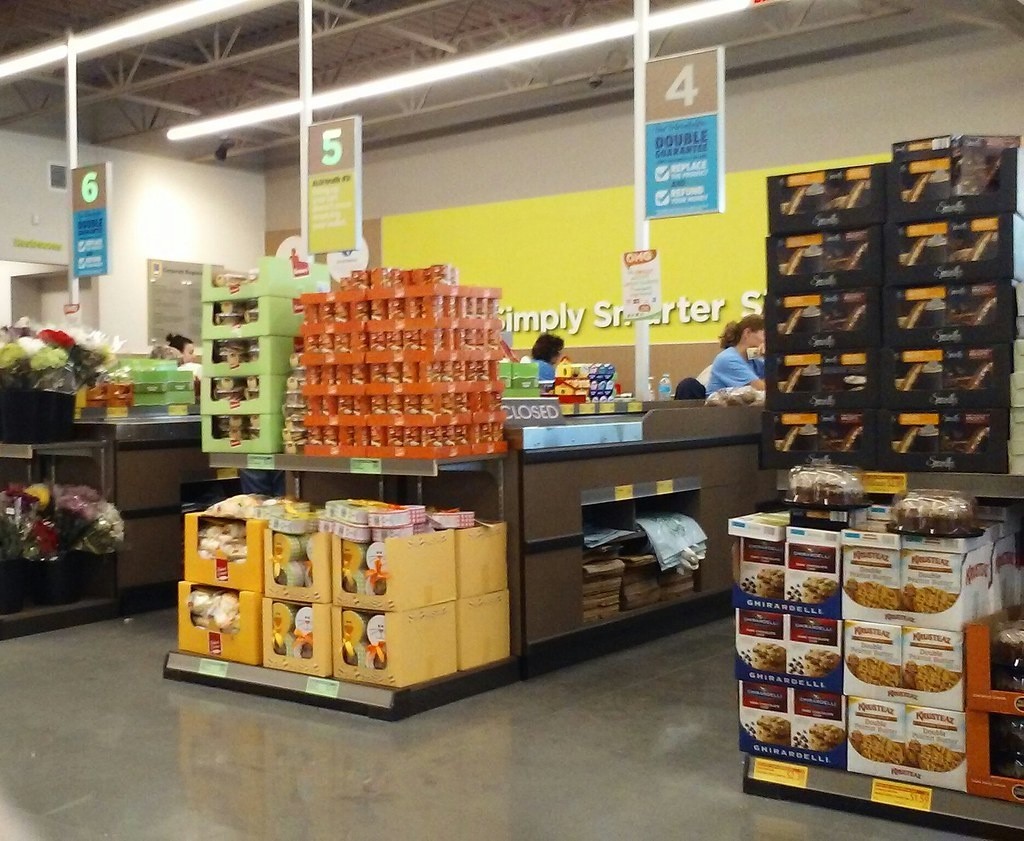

[891,502,976,538]
[789,476,865,507]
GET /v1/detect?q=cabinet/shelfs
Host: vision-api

[0,438,119,640]
[161,446,525,724]
[740,464,1024,841]
[283,401,767,681]
[40,415,209,616]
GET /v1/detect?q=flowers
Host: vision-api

[0,482,125,560]
[0,316,125,394]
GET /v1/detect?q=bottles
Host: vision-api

[648,377,655,402]
[658,374,671,401]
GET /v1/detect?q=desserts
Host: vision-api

[750,569,844,753]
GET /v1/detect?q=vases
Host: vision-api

[36,391,76,441]
[0,390,41,443]
[0,559,32,615]
[29,557,78,605]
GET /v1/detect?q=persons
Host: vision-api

[239,468,286,497]
[166,333,196,362]
[532,334,565,364]
[705,314,765,397]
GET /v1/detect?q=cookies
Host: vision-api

[850,582,963,774]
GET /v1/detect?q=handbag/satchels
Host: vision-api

[634,510,708,575]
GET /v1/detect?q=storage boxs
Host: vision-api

[106,257,618,686]
[729,136,1024,803]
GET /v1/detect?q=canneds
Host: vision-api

[306,265,504,449]
